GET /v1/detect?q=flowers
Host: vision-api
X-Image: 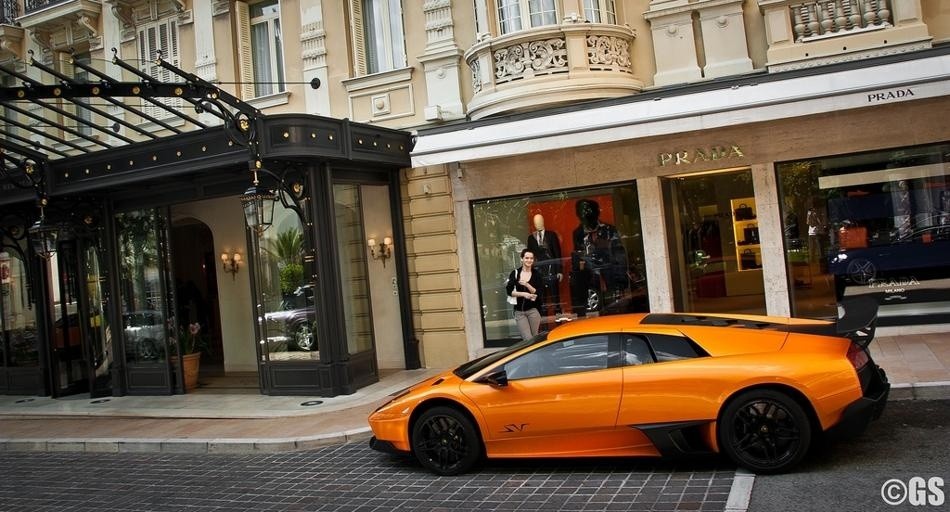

[165,316,200,355]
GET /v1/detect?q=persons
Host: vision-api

[896,180,911,217]
[572,197,631,292]
[805,194,827,263]
[505,249,545,341]
[527,213,565,317]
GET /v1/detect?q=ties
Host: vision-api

[538,230,543,245]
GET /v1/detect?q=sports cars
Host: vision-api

[366,296,892,477]
[601,255,813,317]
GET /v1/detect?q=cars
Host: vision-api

[0,309,176,368]
[481,254,630,321]
[826,210,950,288]
[256,306,319,353]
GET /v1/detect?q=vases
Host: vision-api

[167,351,202,390]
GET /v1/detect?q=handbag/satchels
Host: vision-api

[744,227,759,244]
[506,278,518,305]
[741,253,756,270]
[735,207,753,221]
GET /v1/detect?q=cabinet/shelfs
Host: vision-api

[729,197,762,271]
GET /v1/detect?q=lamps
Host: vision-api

[238,170,280,237]
[423,105,452,122]
[367,237,392,268]
[221,253,241,282]
[28,207,60,261]
